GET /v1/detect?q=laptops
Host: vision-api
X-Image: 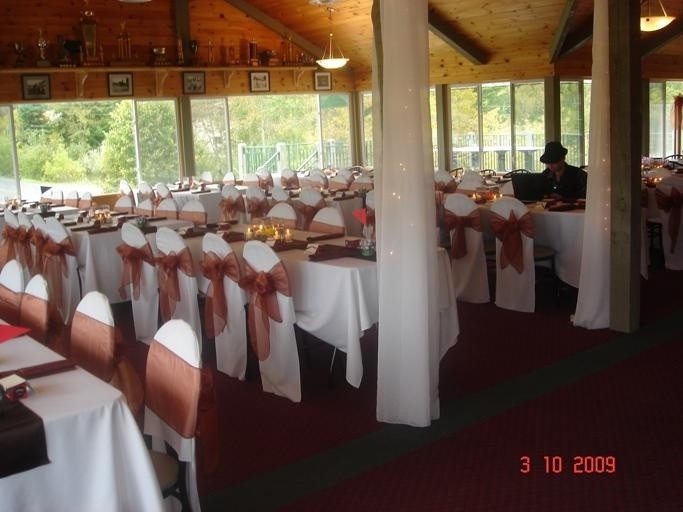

[510,173,551,204]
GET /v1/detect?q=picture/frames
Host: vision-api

[180,71,205,95]
[312,72,332,92]
[248,70,269,94]
[106,73,133,96]
[19,74,51,100]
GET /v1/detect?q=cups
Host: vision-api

[245,223,294,245]
[96,209,111,224]
[471,191,503,204]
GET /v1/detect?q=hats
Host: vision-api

[540,140,568,164]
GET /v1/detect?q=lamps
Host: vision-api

[638,1,674,34]
[313,7,351,71]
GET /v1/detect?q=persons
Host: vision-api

[539,141,588,203]
